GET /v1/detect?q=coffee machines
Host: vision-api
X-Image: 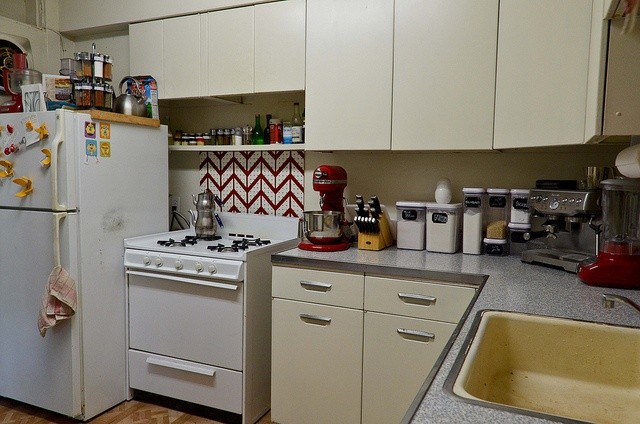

[521,187,603,275]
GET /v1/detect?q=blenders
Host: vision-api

[576,178,640,290]
[0,52,43,113]
[297,165,353,252]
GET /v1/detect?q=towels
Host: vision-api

[36,265,78,338]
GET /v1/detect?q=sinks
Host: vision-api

[453,312,639,423]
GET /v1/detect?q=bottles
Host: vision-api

[264,114,272,144]
[510,188,531,224]
[482,237,507,256]
[508,224,532,255]
[168,129,211,146]
[292,103,303,144]
[82,51,92,77]
[92,53,105,78]
[94,83,105,107]
[105,84,112,108]
[486,188,510,238]
[210,129,216,145]
[251,114,265,144]
[243,126,253,145]
[235,127,242,145]
[103,55,112,80]
[82,82,93,106]
[217,128,224,145]
[74,51,82,77]
[462,186,486,255]
[230,128,235,145]
[75,82,83,106]
[224,128,230,145]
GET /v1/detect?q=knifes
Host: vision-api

[367,200,378,214]
[353,216,379,233]
[354,200,369,216]
[371,195,381,213]
[355,194,364,209]
[370,208,380,220]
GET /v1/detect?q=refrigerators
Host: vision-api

[0,109,169,422]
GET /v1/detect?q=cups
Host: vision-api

[584,166,617,191]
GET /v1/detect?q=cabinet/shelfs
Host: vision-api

[206,0,307,96]
[128,13,205,100]
[128,347,244,416]
[271,264,365,424]
[304,0,640,152]
[361,271,479,424]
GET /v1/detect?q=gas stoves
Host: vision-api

[123,229,297,282]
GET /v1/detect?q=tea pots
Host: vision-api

[187,208,217,237]
[111,76,147,117]
[191,189,222,211]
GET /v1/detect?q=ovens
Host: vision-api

[126,264,271,424]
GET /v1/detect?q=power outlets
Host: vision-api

[170,195,181,212]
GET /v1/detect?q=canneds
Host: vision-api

[189,132,196,145]
[182,132,189,145]
[196,133,204,145]
[204,133,210,145]
[174,130,182,146]
[270,118,284,144]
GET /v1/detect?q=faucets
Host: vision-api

[600,291,639,320]
